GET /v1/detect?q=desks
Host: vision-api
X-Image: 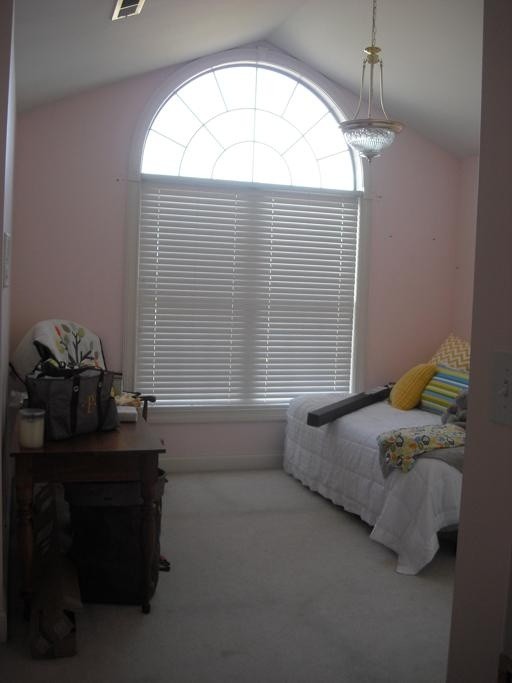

[7,413,167,623]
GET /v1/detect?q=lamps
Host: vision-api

[338,0,405,167]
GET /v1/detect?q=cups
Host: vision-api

[17,407,46,449]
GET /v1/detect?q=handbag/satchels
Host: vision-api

[25,356,122,441]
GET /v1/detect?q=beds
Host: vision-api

[282,391,463,576]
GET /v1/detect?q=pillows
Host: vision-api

[389,332,471,416]
